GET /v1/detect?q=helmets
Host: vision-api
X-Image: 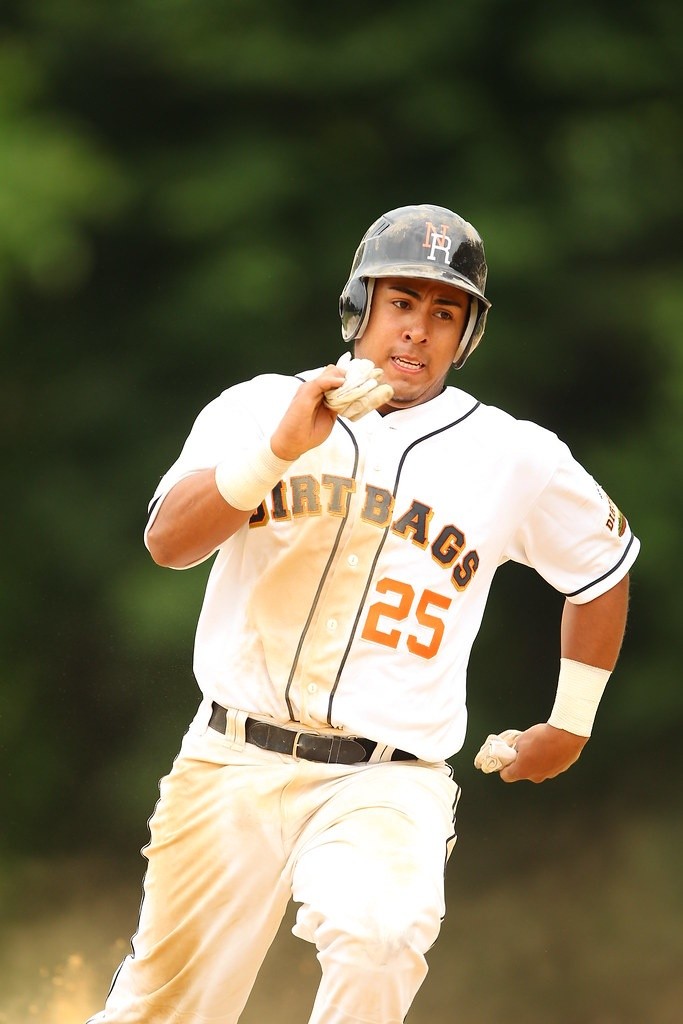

[337,204,492,372]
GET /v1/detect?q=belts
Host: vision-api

[208,702,418,766]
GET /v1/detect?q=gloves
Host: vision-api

[474,728,524,775]
[327,351,395,422]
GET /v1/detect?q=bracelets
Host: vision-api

[548,659,614,736]
[215,439,296,509]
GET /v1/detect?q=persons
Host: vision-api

[90,204,642,1024]
